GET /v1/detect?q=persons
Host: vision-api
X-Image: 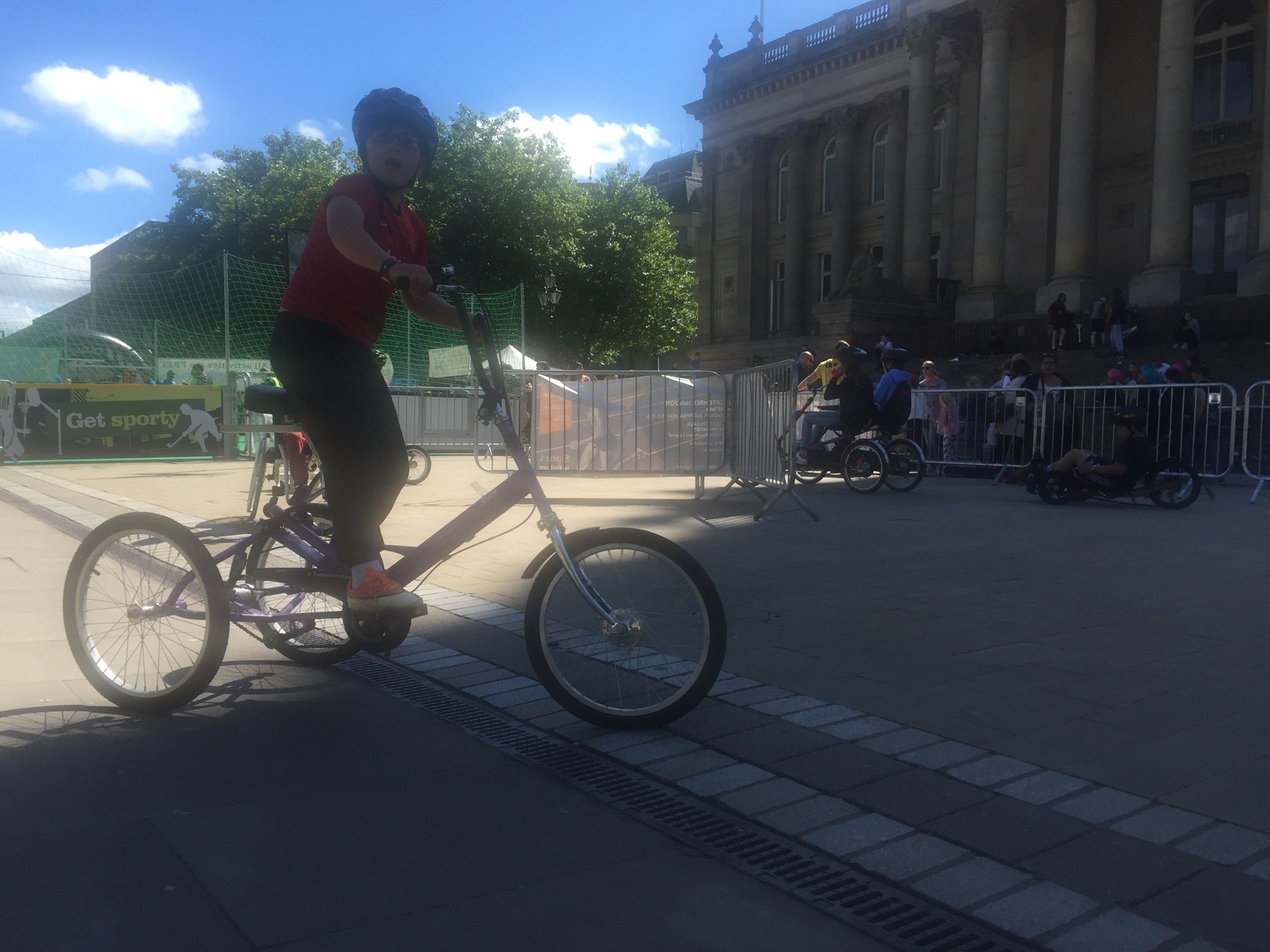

[267,87,490,613]
[794,347,868,467]
[1172,311,1200,351]
[0,292,1270,505]
[938,393,958,473]
[1032,412,1149,498]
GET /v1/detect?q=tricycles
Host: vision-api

[1027,455,1201,511]
[63,261,729,734]
[731,382,924,493]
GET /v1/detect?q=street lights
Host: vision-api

[538,268,562,380]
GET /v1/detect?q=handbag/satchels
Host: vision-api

[1047,402,1074,427]
[986,422,998,447]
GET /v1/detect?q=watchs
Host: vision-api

[1092,465,1097,474]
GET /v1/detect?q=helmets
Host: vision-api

[837,347,867,362]
[351,87,439,167]
[1110,406,1147,428]
[879,347,908,362]
[373,350,387,365]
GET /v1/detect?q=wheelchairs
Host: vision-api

[245,433,325,520]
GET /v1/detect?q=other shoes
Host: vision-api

[781,452,808,466]
[1052,345,1069,352]
[1173,343,1178,350]
[288,485,308,506]
[1023,459,1048,485]
[1109,353,1125,358]
[1179,343,1189,350]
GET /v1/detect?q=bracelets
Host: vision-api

[378,255,401,282]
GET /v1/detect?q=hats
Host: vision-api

[1010,353,1025,363]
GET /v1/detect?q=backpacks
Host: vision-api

[884,380,912,429]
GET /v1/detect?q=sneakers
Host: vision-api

[347,567,428,619]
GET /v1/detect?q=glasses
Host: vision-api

[802,356,814,363]
[368,128,425,149]
[1041,352,1057,358]
[920,366,931,370]
